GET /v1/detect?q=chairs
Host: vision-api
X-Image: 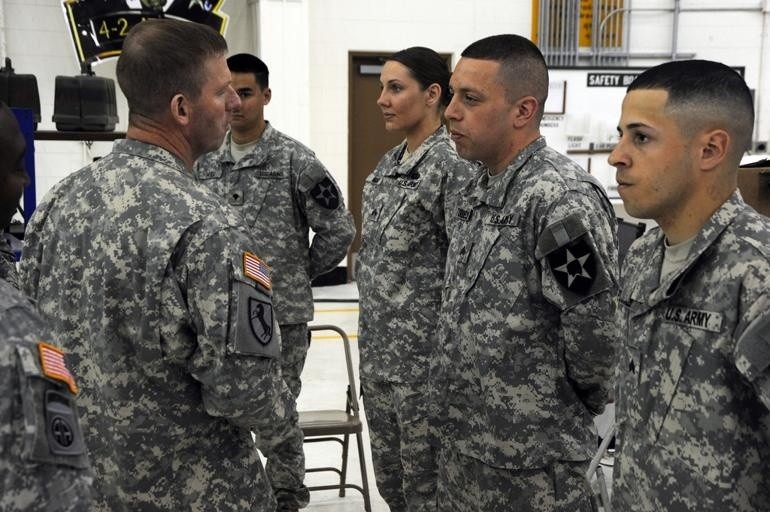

[297,325,374,512]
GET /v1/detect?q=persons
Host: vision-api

[354,48,485,512]
[1,100,101,511]
[193,52,357,511]
[15,16,299,510]
[608,58,769,512]
[424,35,624,512]
